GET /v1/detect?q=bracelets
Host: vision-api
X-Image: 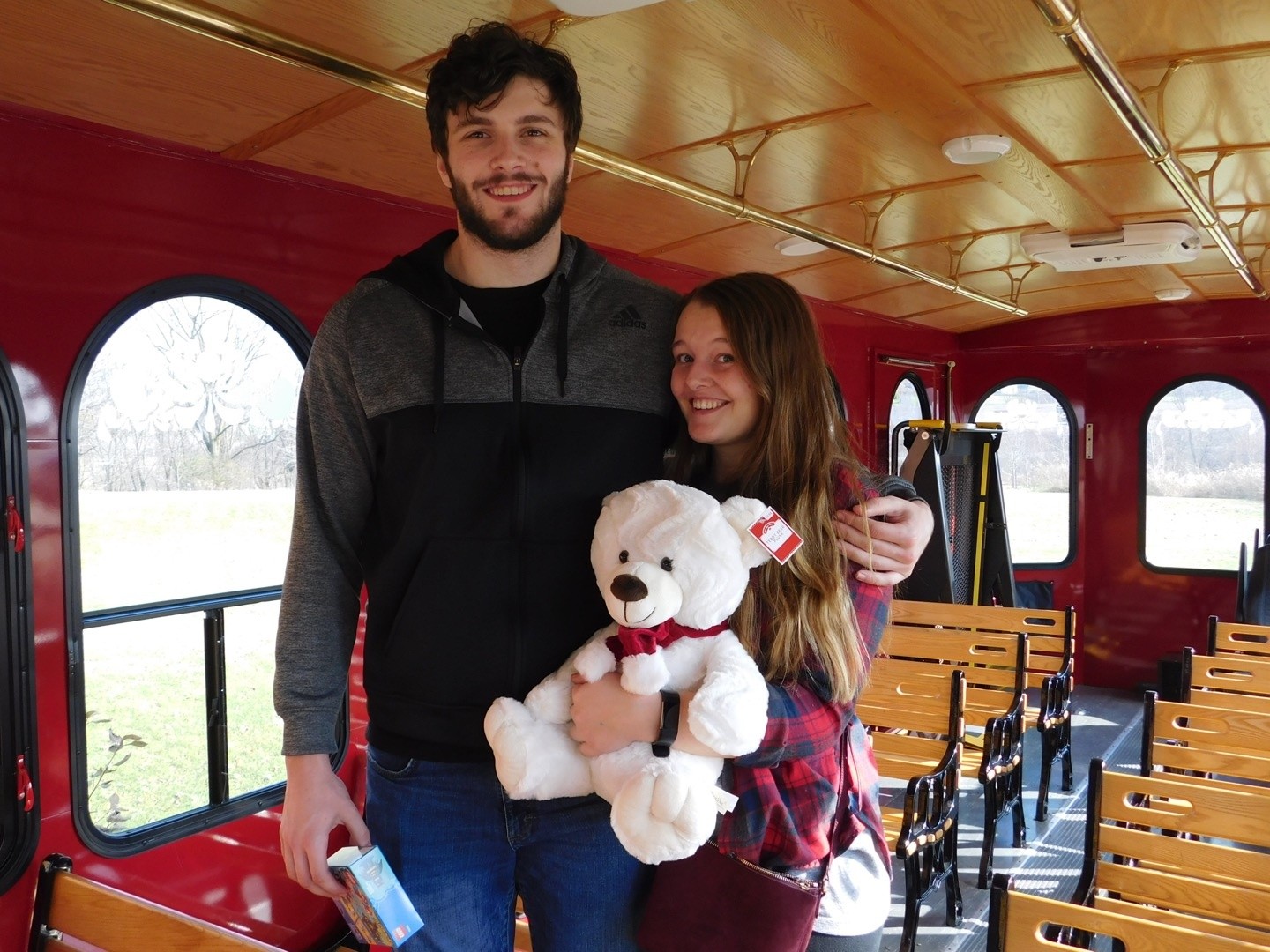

[651,690,682,757]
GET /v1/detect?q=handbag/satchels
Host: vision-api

[635,839,824,951]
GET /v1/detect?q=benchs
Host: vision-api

[855,596,1267,952]
[29,853,300,951]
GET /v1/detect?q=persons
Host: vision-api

[563,270,897,952]
[261,12,941,952]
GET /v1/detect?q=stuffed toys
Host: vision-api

[482,478,786,869]
